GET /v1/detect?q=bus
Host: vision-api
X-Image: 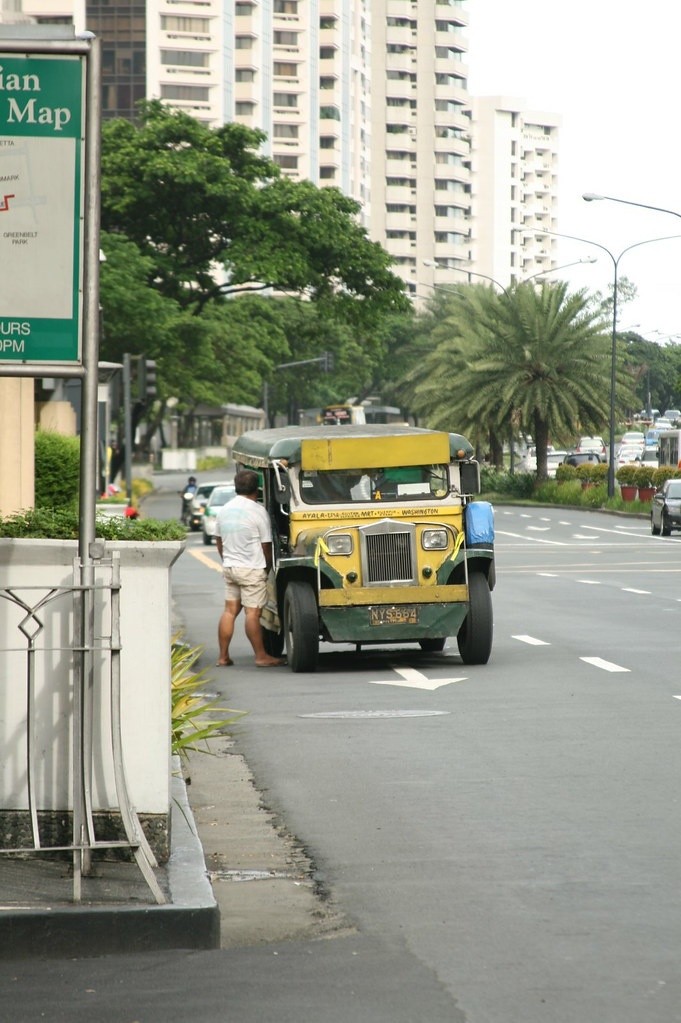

[315,403,367,426]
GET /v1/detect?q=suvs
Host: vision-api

[231,419,497,671]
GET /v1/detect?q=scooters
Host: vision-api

[175,490,196,529]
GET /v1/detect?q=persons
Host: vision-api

[181,477,197,520]
[215,471,288,667]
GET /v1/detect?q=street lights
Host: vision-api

[418,254,602,301]
[514,222,681,500]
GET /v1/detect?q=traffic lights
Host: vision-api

[138,356,157,404]
[324,351,335,373]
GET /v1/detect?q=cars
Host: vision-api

[187,480,233,529]
[649,479,681,536]
[199,486,235,545]
[521,409,681,478]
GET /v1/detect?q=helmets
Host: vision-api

[189,477,196,486]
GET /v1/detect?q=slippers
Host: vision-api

[257,658,288,667]
[215,658,233,667]
